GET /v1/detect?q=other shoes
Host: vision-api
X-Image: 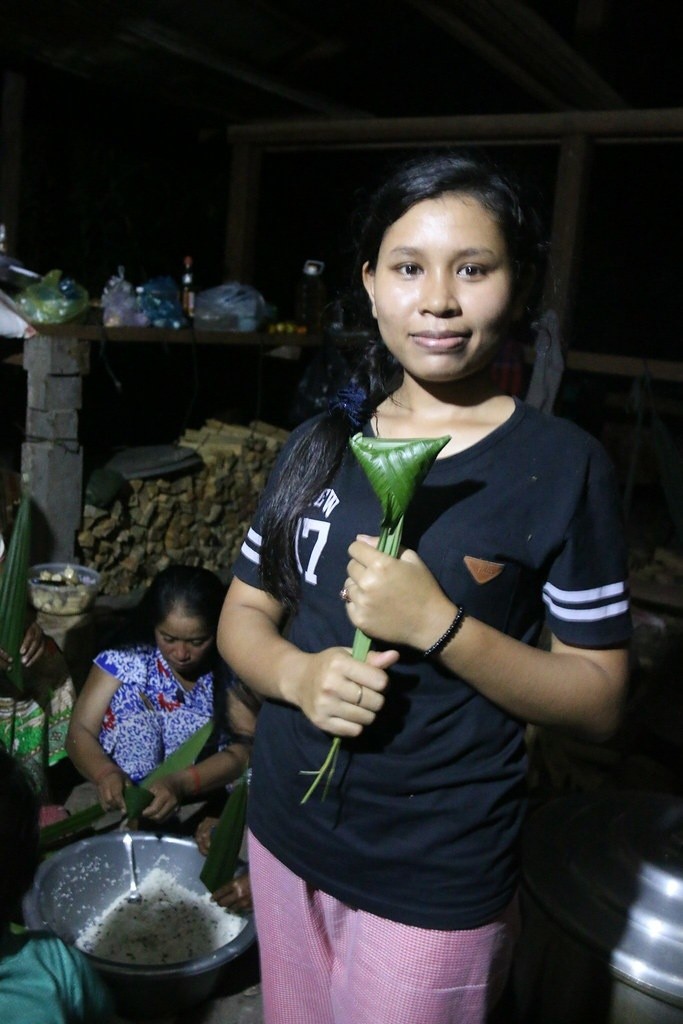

[38,803,67,826]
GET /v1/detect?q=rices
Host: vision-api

[73,866,243,966]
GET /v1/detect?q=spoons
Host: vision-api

[123,833,142,905]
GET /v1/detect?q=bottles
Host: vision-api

[181,255,197,324]
[331,290,349,330]
[135,287,150,323]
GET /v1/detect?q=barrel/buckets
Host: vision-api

[287,259,325,330]
[512,791,682,1023]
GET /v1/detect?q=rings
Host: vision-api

[356,684,363,706]
[340,587,351,601]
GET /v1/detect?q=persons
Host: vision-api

[0,750,122,1024]
[0,535,46,672]
[192,666,262,917]
[65,564,252,826]
[213,161,633,1023]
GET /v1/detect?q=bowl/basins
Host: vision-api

[26,563,101,615]
[22,830,257,1010]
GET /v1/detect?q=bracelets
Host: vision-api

[423,603,466,659]
[187,767,204,795]
